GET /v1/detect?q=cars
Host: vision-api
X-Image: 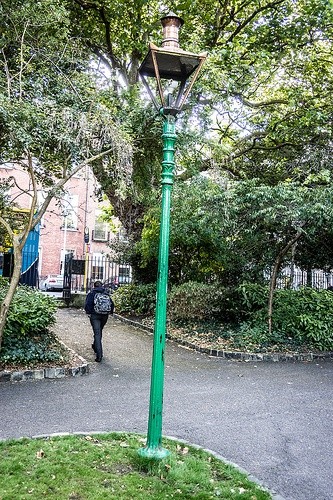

[38,274,66,291]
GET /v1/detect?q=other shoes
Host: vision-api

[94,357,101,362]
[91,343,96,352]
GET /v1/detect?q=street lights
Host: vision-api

[135,10,210,475]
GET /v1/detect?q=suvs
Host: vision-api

[102,276,132,289]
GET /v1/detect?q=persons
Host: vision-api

[84,281,114,362]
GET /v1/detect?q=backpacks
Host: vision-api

[94,293,112,313]
